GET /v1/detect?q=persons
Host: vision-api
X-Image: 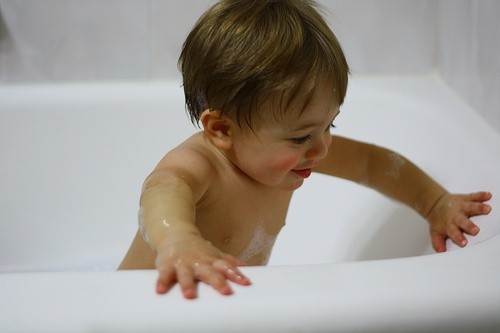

[115,0,492,301]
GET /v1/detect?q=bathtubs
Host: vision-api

[0,72,500,332]
